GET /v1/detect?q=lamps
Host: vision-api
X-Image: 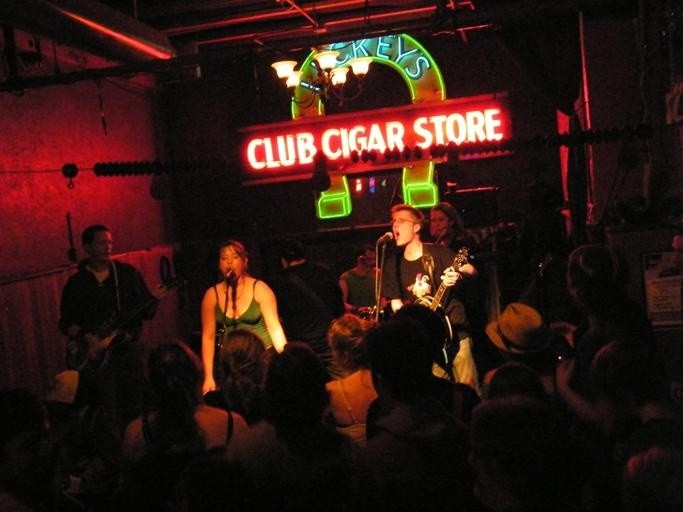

[267,42,375,111]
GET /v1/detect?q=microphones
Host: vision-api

[374,231,393,246]
[224,265,235,279]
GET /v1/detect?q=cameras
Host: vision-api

[543,332,569,362]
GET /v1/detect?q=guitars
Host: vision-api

[63,272,191,385]
[413,245,469,314]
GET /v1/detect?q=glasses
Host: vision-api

[389,217,416,226]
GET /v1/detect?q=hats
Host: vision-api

[483,301,554,357]
[42,368,98,405]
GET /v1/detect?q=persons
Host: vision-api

[2,195,683,511]
